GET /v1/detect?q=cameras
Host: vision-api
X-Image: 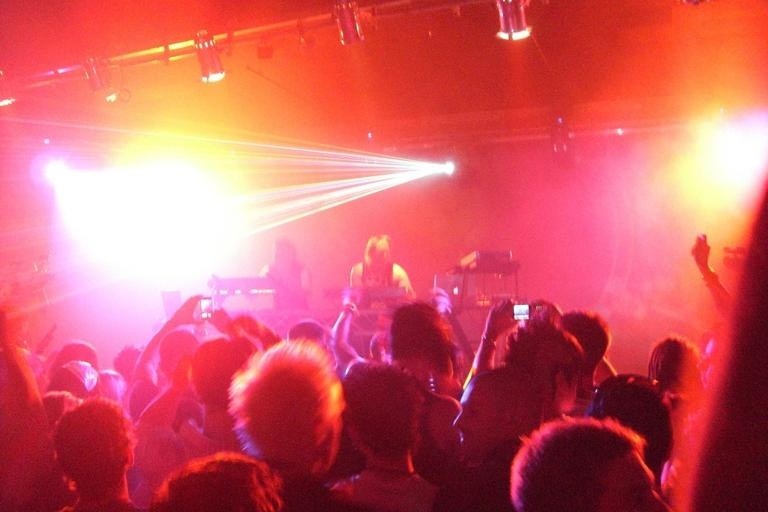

[201,294,215,320]
[512,301,530,322]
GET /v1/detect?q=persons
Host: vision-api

[1,230,768,512]
